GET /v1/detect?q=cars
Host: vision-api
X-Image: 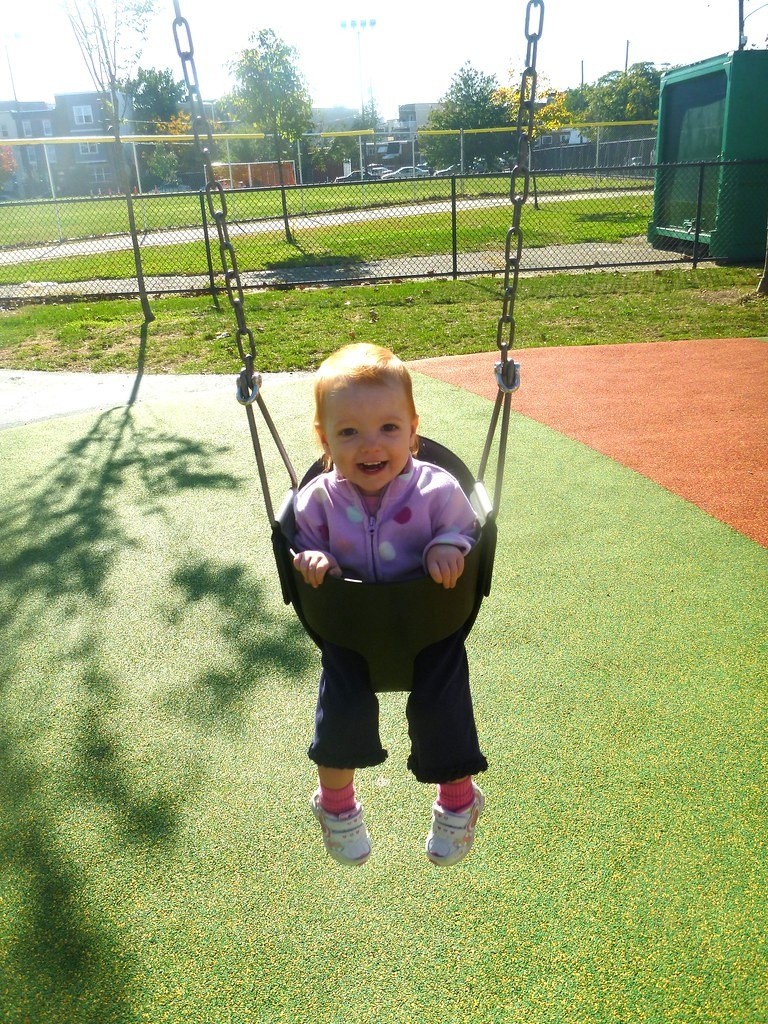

[367,162,474,179]
[335,170,381,182]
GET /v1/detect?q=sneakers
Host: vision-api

[425,782,484,865]
[311,790,371,866]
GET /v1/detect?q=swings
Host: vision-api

[169,0,544,688]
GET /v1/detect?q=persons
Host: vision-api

[290,341,490,869]
